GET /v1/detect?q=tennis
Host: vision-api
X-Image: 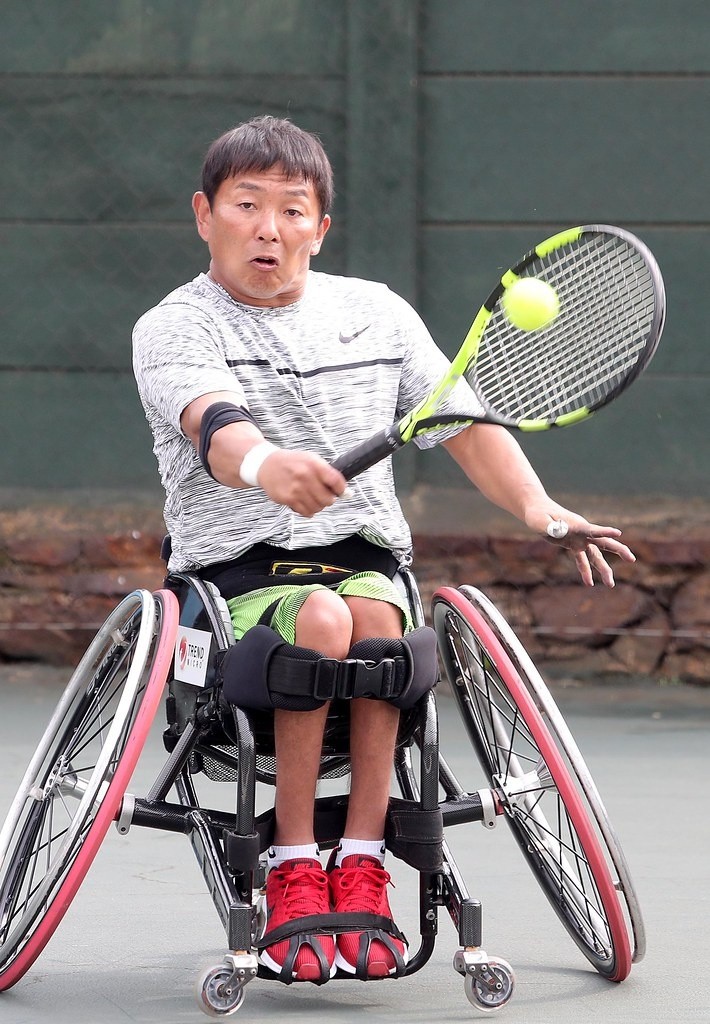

[501,277,560,330]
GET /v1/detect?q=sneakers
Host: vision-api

[256,858,337,980]
[325,845,409,976]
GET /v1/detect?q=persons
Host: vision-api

[132,119,634,986]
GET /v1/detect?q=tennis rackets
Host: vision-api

[331,226,667,480]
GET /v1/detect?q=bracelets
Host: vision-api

[239,441,278,486]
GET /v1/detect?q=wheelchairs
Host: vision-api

[1,536,645,1018]
